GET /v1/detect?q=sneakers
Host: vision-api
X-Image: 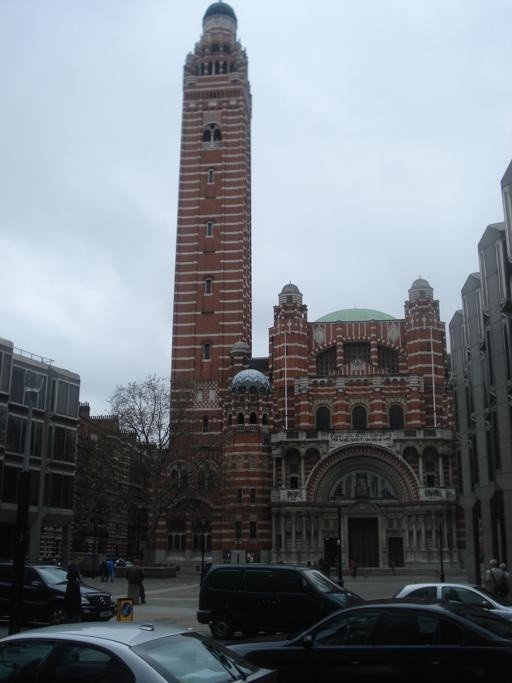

[101,579,113,582]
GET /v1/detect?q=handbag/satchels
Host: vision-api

[495,584,508,596]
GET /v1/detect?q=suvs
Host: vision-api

[196,562,369,640]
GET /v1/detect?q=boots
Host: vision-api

[141,598,145,604]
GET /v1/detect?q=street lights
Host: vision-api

[333,485,346,588]
[198,515,207,586]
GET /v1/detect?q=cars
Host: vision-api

[227,597,512,683]
[1,622,279,683]
[392,582,512,621]
[0,562,119,626]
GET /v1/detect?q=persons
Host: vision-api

[107,557,116,582]
[99,559,108,582]
[60,560,82,623]
[126,560,146,604]
[497,562,512,604]
[484,558,507,604]
